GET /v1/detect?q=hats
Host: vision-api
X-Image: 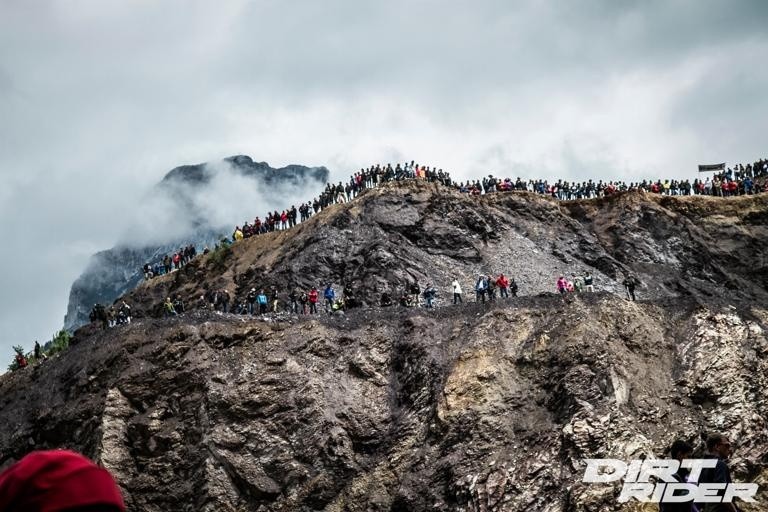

[0,449,125,511]
